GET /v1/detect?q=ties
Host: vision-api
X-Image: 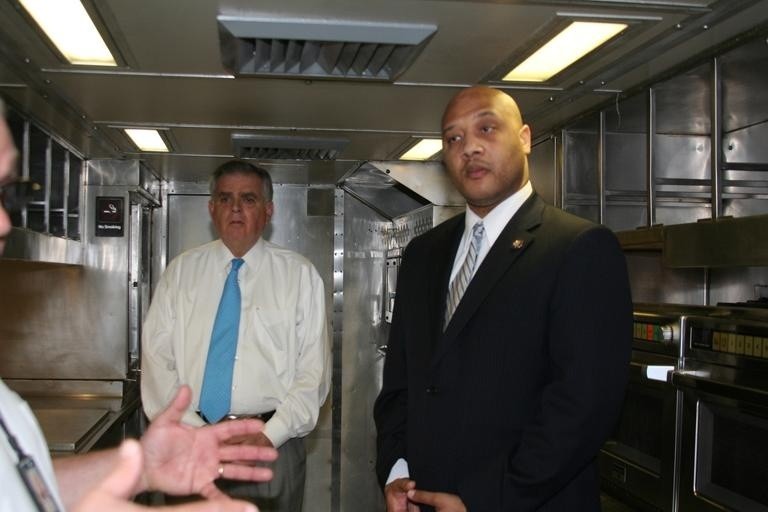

[442,220,485,333]
[198,257,245,424]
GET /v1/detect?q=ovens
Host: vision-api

[595,216,767,512]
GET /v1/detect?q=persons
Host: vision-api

[0,103,280,511]
[138,159,334,511]
[371,86,635,511]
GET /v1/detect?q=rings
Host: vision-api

[217,461,224,477]
